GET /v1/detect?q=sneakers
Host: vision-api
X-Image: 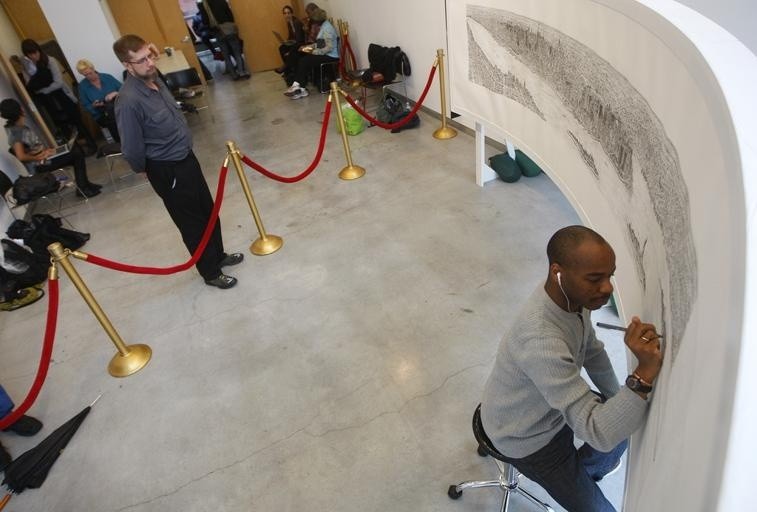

[77,179,102,196]
[2,415,42,436]
[284,85,309,99]
[206,274,237,289]
[220,253,243,267]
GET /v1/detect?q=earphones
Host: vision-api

[556,272,561,286]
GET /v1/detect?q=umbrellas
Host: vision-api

[0,393,103,512]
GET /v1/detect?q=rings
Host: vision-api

[641,337,649,342]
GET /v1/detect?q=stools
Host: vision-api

[446,400,557,512]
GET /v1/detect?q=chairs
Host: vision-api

[166,67,209,118]
[0,170,89,230]
[317,36,341,95]
[348,43,383,101]
[7,146,74,197]
[363,52,409,116]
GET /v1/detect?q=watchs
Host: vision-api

[625,375,652,393]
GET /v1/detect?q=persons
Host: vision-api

[274,3,339,99]
[186,0,251,81]
[0,98,103,197]
[112,34,244,289]
[20,39,97,156]
[76,58,123,143]
[477,225,662,512]
[0,384,43,437]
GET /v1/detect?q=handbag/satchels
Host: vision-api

[12,171,60,205]
[335,103,365,135]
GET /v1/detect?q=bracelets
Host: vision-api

[634,373,653,387]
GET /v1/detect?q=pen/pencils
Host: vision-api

[597,323,663,337]
